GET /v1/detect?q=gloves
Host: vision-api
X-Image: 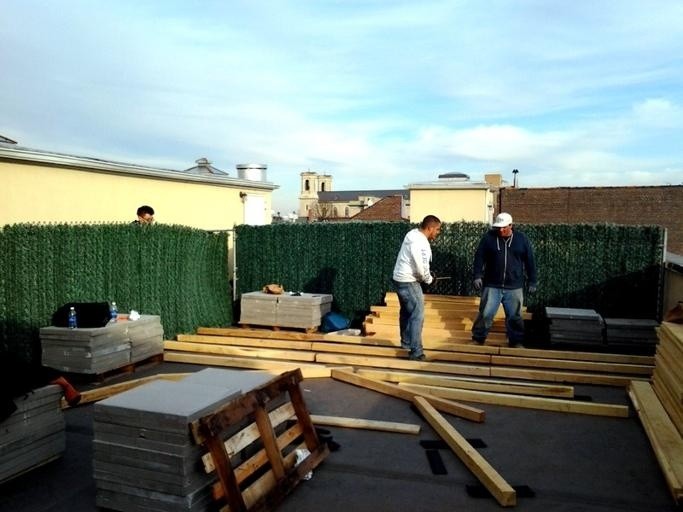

[473,278,483,289]
[528,287,537,293]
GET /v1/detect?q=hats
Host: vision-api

[492,212,513,229]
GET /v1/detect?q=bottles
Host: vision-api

[68,307,76,331]
[110,301,117,322]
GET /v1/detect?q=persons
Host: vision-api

[469,213,538,348]
[391,215,441,362]
[129,205,156,224]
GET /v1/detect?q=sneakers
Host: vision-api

[417,355,426,361]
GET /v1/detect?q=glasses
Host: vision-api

[142,217,154,222]
